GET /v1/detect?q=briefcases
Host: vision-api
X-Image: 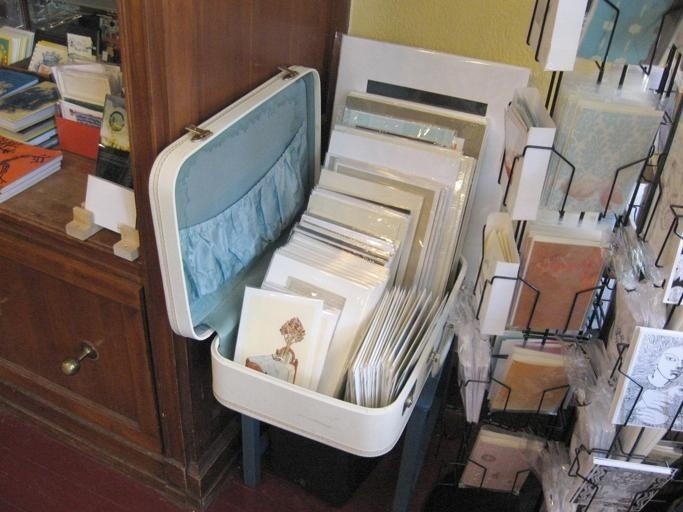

[148,64,468,458]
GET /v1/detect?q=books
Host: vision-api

[0,18,65,207]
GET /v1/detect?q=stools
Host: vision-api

[240,363,451,512]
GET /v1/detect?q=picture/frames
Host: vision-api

[329,31,531,293]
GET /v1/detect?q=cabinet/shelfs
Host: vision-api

[0,0,338,512]
